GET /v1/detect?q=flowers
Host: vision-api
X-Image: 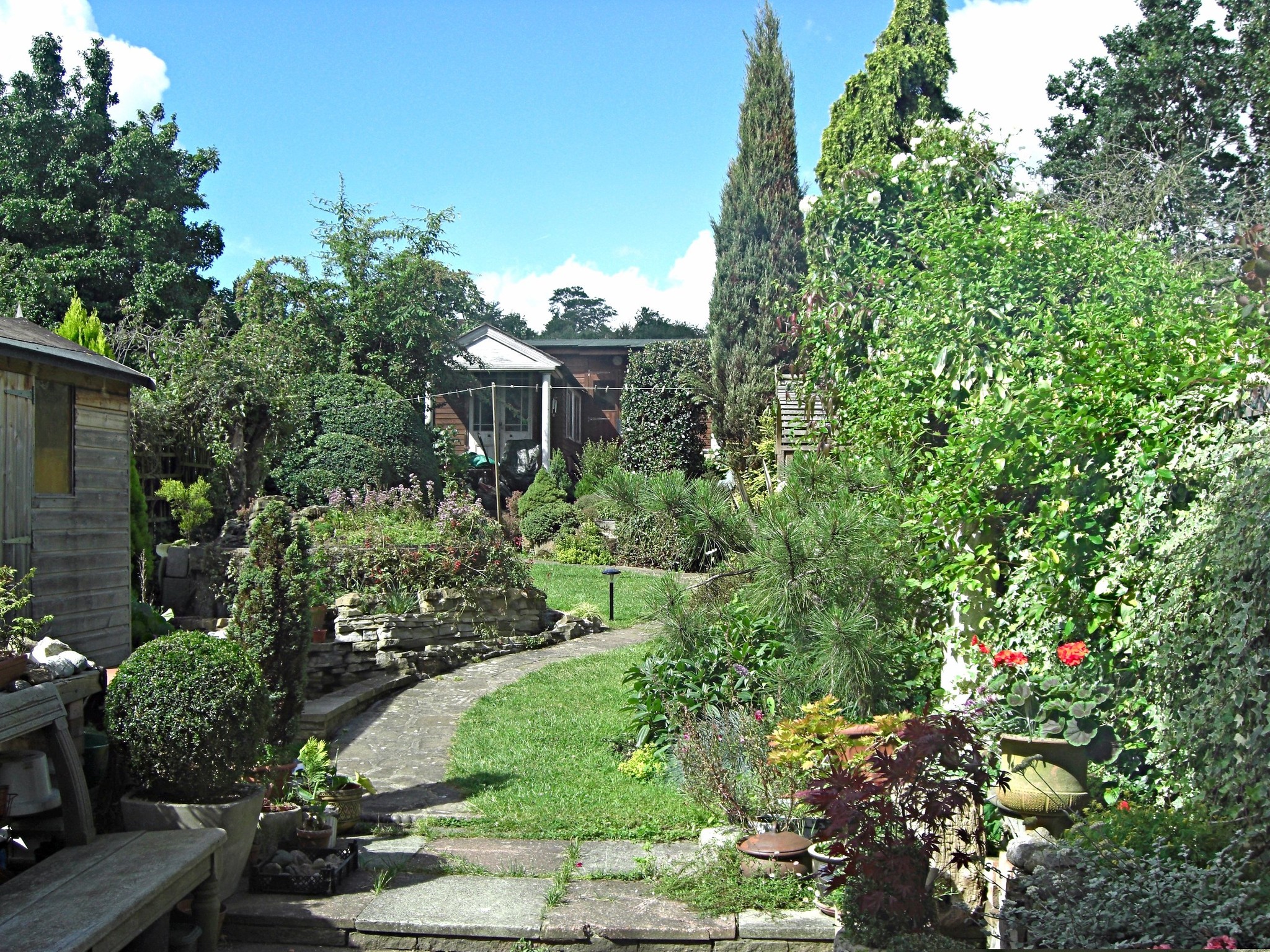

[960,632,1121,764]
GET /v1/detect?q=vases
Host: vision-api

[990,729,1089,820]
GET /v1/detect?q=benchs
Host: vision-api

[0,680,228,952]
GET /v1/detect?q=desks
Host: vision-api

[0,668,103,817]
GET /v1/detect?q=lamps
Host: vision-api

[601,567,622,584]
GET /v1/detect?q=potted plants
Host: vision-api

[96,473,376,879]
[809,832,857,913]
